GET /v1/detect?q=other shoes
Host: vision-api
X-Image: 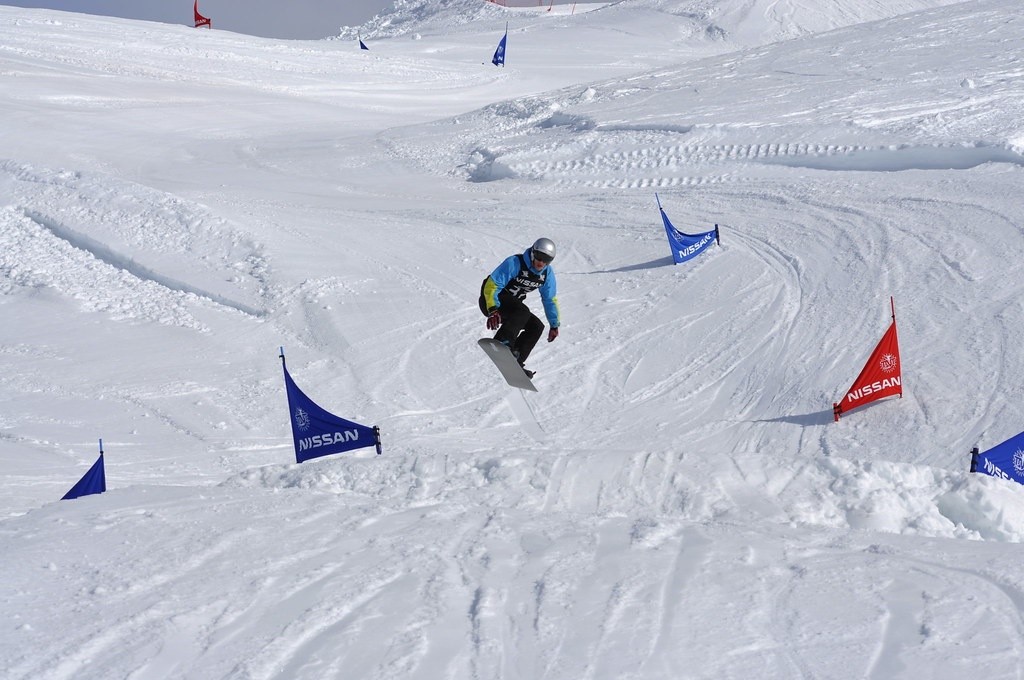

[518,362,533,379]
[512,350,520,359]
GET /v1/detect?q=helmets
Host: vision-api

[533,238,556,260]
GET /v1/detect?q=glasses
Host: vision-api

[533,250,553,263]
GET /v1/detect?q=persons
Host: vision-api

[479,238,561,379]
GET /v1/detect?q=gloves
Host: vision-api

[547,328,558,342]
[487,310,501,330]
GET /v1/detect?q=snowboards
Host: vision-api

[478,337,539,394]
